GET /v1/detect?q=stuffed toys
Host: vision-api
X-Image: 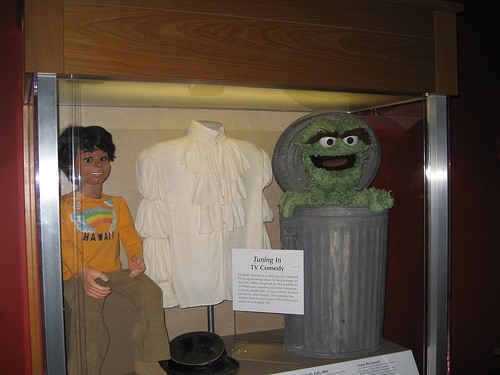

[278,117,396,219]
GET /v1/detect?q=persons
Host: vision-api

[55,124,171,375]
